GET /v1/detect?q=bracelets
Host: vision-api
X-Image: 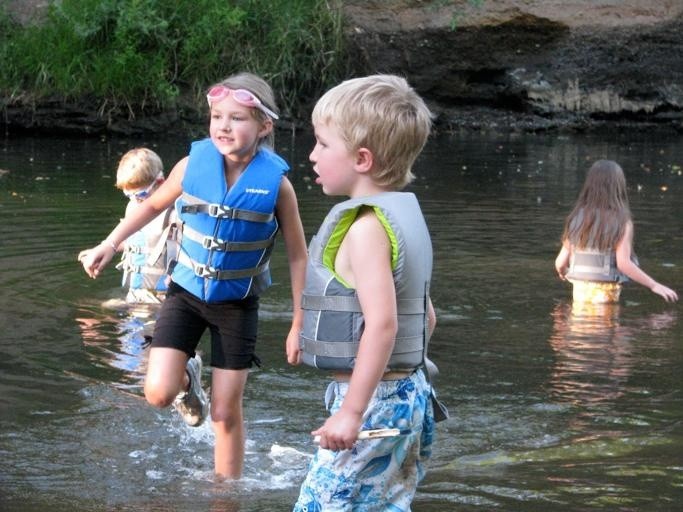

[107,239,119,254]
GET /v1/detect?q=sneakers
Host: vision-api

[173,354,208,428]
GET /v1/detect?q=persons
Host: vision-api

[553,159,678,306]
[290,73,447,512]
[78,73,308,479]
[114,149,179,304]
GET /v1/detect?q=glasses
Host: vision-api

[205,86,280,122]
[122,171,164,199]
